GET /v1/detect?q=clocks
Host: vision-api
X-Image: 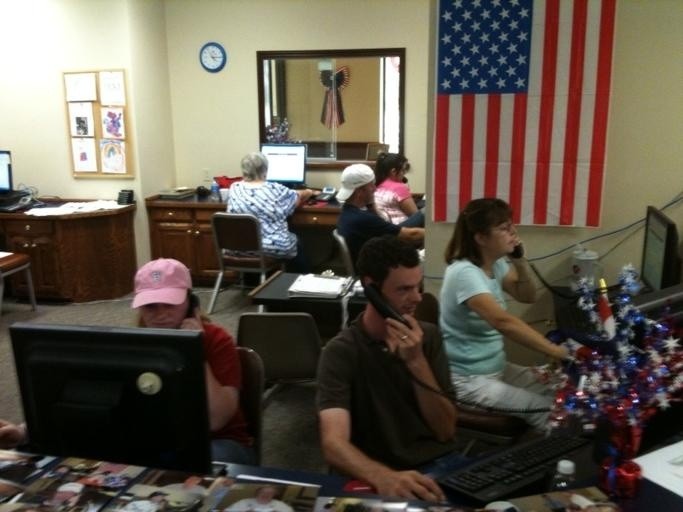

[199,43,228,73]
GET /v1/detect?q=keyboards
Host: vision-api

[438,434,588,502]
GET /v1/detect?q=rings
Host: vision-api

[401,335,408,341]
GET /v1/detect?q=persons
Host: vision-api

[334,163,425,274]
[0,256,253,462]
[315,234,514,505]
[223,152,321,286]
[360,151,419,226]
[439,198,569,437]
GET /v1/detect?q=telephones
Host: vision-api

[0,197,32,212]
[511,245,524,259]
[314,187,337,202]
[365,283,413,331]
[186,294,200,318]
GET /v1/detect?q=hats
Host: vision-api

[132,257,192,309]
[335,163,375,201]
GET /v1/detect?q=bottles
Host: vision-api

[548,459,579,512]
[210,180,220,202]
[570,249,606,293]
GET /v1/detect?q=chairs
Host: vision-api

[232,345,267,468]
[236,311,322,410]
[0,253,37,312]
[413,291,531,459]
[331,229,356,279]
[206,212,287,317]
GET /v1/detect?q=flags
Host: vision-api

[431,0,618,229]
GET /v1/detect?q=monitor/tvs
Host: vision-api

[640,205,679,292]
[259,143,307,187]
[0,150,14,192]
[8,322,212,476]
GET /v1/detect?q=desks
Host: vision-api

[373,283,682,512]
[2,443,484,512]
[1,196,138,301]
[248,268,367,336]
[144,189,342,286]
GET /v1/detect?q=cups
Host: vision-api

[219,187,229,201]
